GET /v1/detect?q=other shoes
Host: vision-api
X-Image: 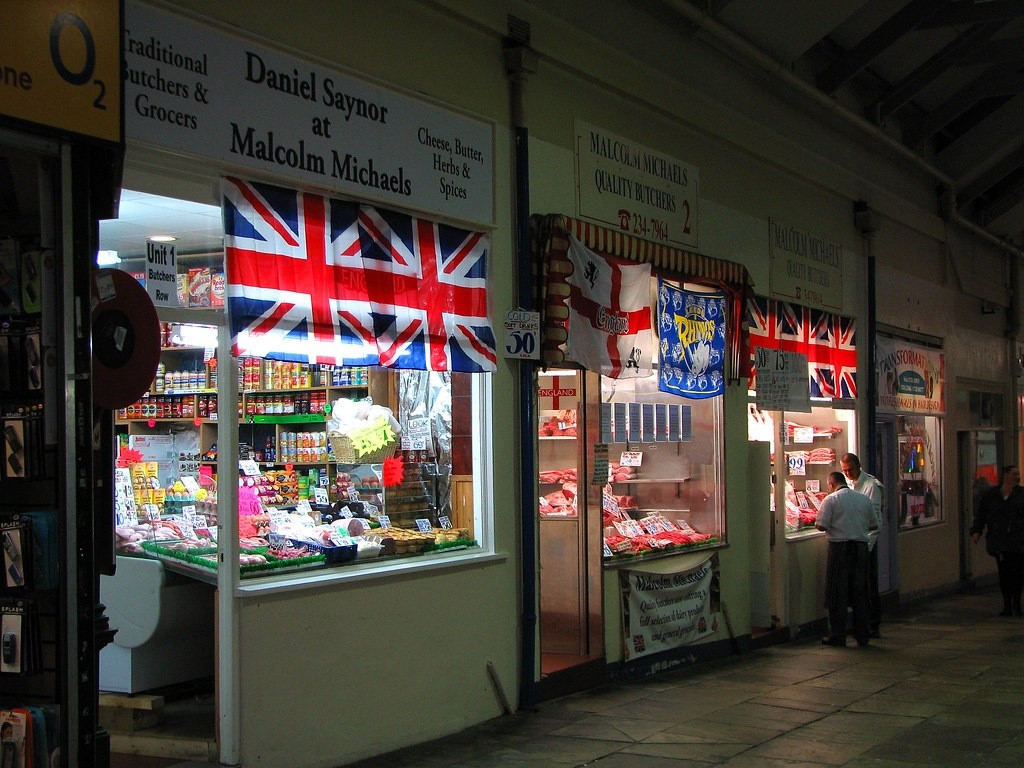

[998,610,1014,617]
[857,637,868,646]
[822,635,847,647]
[1015,610,1024,617]
[870,630,881,637]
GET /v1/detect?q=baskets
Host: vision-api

[327,430,402,464]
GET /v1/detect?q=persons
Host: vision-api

[814,471,879,646]
[839,453,883,638]
[770,456,796,511]
[970,465,1024,617]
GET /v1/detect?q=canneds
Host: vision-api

[198,392,326,418]
[264,435,276,462]
[280,431,327,463]
[244,356,312,389]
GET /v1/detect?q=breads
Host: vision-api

[364,524,470,554]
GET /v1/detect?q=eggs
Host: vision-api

[166,485,217,502]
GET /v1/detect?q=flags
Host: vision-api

[657,277,726,399]
[357,200,498,372]
[562,234,654,379]
[218,175,399,369]
[748,296,858,399]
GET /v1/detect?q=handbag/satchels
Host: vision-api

[985,531,1010,555]
